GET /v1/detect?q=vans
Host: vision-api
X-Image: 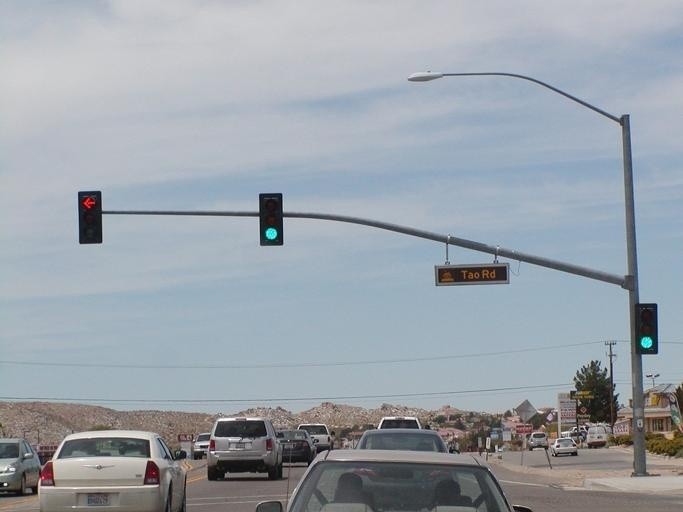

[527,431,549,451]
[586,425,609,449]
[377,415,423,430]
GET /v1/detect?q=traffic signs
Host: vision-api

[434,264,510,286]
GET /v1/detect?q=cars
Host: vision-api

[274,429,319,466]
[561,424,587,443]
[551,435,580,457]
[0,436,43,498]
[34,427,190,512]
[350,428,457,488]
[253,444,535,512]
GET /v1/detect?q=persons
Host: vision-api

[420,480,462,512]
[331,473,377,512]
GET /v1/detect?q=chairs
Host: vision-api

[319,503,374,512]
[430,505,480,512]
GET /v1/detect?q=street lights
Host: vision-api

[646,372,660,387]
[405,70,652,475]
[35,428,40,444]
[23,429,31,438]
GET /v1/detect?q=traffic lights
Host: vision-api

[77,190,103,245]
[636,302,658,355]
[260,193,283,247]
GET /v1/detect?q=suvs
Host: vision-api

[207,415,285,483]
[296,424,335,451]
[193,431,212,460]
